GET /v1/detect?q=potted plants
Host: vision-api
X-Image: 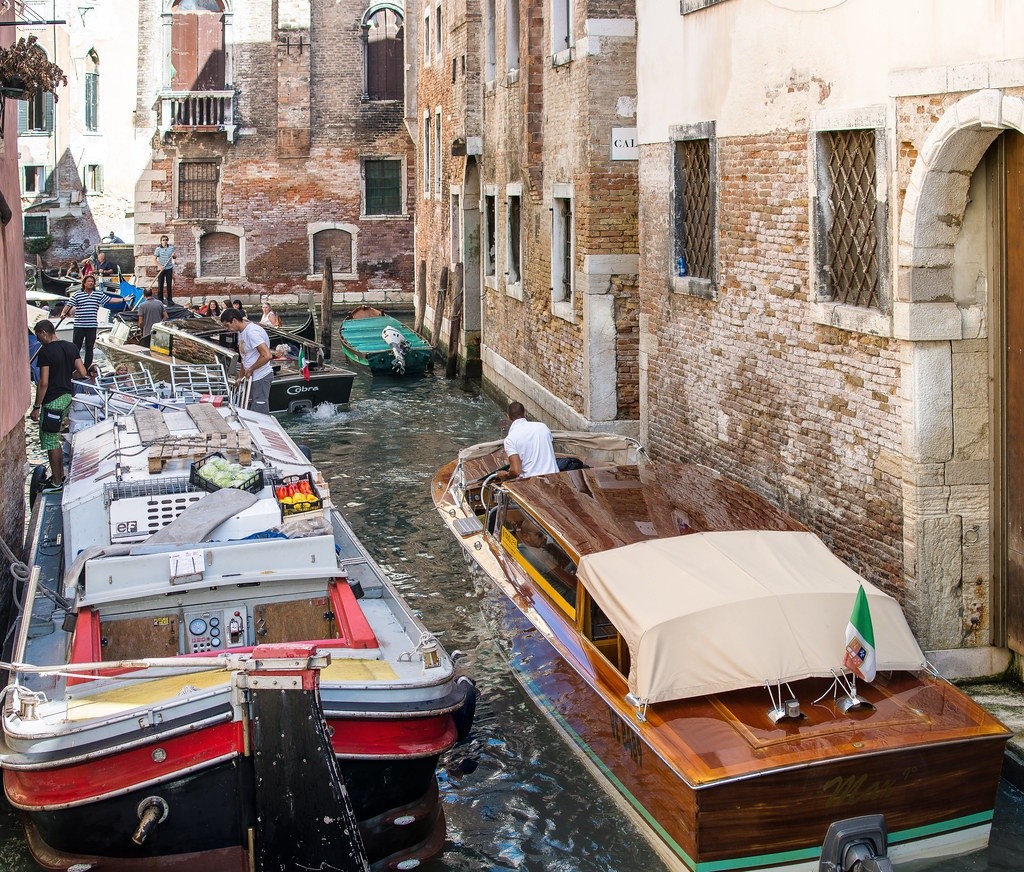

[0,35,68,103]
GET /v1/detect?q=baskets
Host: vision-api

[271,472,323,524]
[189,451,264,494]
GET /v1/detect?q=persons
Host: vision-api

[30,319,87,495]
[137,288,169,348]
[109,231,124,243]
[184,300,246,317]
[60,275,136,370]
[73,363,137,395]
[153,234,177,306]
[497,401,560,480]
[259,303,276,327]
[517,519,599,618]
[220,308,274,415]
[66,253,113,278]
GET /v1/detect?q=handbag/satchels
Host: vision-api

[42,407,63,433]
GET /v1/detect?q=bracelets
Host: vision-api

[33,405,40,410]
[123,298,124,302]
[104,271,105,273]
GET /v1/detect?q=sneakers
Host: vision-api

[35,483,63,494]
[39,475,67,486]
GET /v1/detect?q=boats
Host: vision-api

[431,431,1014,872]
[26,266,135,319]
[0,290,476,862]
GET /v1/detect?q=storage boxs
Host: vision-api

[189,451,264,494]
[272,471,322,522]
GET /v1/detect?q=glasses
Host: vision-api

[94,370,101,373]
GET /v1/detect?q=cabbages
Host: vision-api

[198,458,258,489]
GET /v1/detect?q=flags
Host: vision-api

[297,347,311,381]
[844,583,877,683]
